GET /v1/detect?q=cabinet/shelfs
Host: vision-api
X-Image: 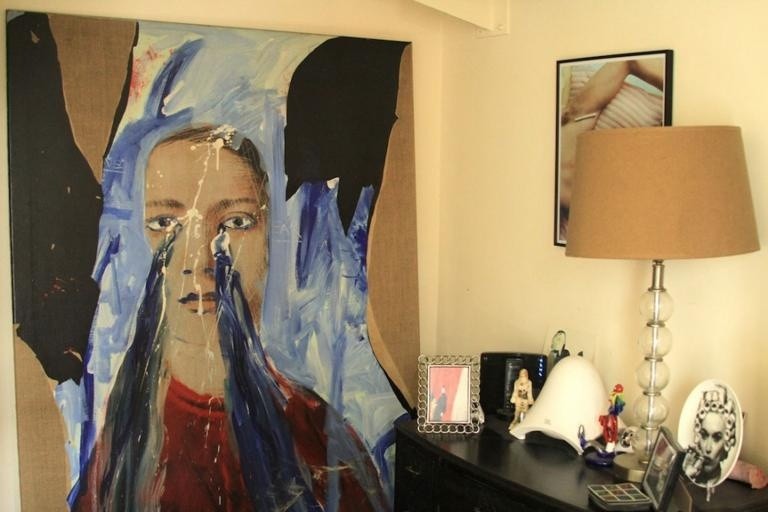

[395,407,768,512]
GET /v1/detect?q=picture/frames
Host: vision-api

[555,49,673,246]
[417,355,481,434]
[642,427,686,511]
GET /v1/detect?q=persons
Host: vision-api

[562,60,667,213]
[72,122,389,512]
[507,367,534,430]
[431,388,447,421]
[682,386,738,485]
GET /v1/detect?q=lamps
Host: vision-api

[567,128,761,481]
